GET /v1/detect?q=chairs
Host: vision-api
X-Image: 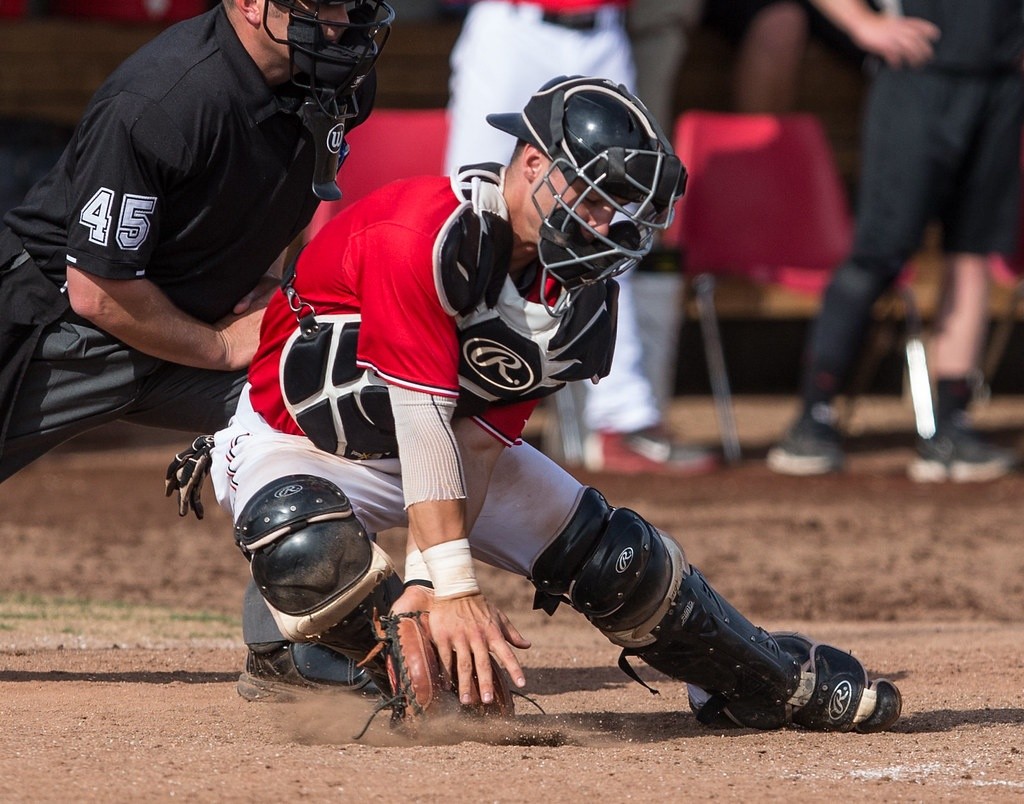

[309,108,935,475]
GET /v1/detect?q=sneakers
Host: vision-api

[238,643,384,703]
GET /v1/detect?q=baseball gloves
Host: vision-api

[381,611,515,738]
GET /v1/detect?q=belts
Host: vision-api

[542,13,592,28]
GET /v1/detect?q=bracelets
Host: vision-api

[424,537,480,599]
[403,551,431,588]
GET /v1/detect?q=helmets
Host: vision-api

[486,74,662,200]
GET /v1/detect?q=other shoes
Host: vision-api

[908,432,1013,484]
[583,430,717,477]
[767,419,839,473]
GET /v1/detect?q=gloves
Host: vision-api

[166,437,214,520]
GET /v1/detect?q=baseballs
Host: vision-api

[435,688,460,725]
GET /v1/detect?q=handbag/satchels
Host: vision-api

[0,230,69,451]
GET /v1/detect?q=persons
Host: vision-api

[209,74,900,732]
[767,0,1024,482]
[0,0,396,483]
[449,1,714,472]
